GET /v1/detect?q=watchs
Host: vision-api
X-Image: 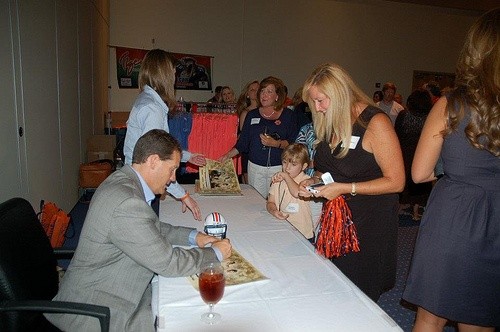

[350,181,357,196]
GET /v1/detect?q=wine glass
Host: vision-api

[199,262,226,323]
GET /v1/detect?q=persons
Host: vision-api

[266,143,323,240]
[302,63,405,303]
[42,128,231,332]
[219,76,298,198]
[238,76,463,218]
[122,48,199,220]
[207,84,223,112]
[402,4,500,332]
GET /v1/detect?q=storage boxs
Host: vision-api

[87,134,116,162]
[80,162,110,188]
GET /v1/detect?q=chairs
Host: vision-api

[0,196,110,332]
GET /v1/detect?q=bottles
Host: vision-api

[192,103,198,114]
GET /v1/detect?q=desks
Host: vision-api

[150,182,404,332]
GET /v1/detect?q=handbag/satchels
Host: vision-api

[37,202,75,250]
[79,160,112,188]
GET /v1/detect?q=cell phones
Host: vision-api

[305,182,325,192]
